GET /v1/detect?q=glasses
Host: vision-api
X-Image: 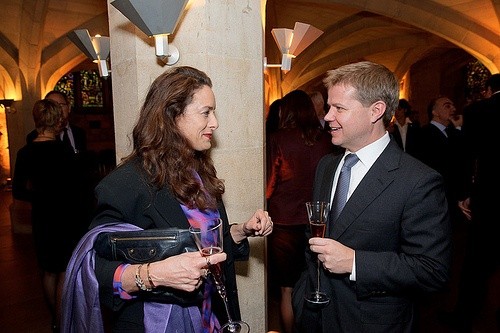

[59,103,68,107]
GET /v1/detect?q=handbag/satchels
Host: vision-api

[93,228,205,308]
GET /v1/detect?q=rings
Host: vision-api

[205,257,210,266]
[195,277,201,286]
[202,269,211,279]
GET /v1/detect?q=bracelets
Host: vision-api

[136,263,151,291]
[146,263,156,289]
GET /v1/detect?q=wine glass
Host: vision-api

[304,200,330,304]
[188,217,250,333]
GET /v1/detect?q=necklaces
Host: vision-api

[38,136,56,139]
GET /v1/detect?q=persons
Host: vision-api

[89,66,273,333]
[268,90,344,333]
[304,61,450,333]
[392,73,500,333]
[12,90,87,333]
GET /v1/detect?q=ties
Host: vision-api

[329,153,360,230]
[62,127,71,146]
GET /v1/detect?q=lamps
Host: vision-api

[263,22,323,73]
[65,28,111,80]
[110,0,188,66]
